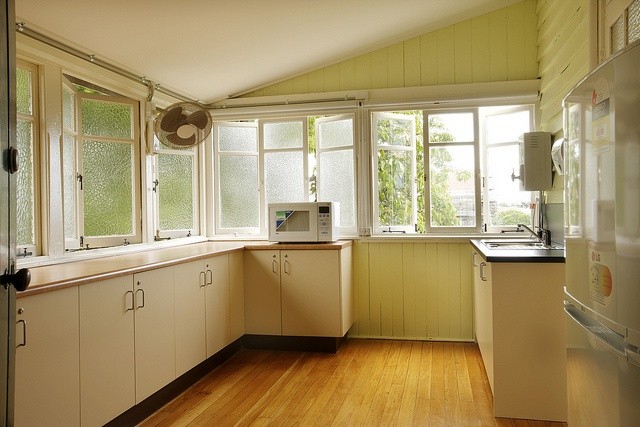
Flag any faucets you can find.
[516,222,553,246]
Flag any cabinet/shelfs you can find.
[471,250,566,422]
[15,285,80,426]
[73,266,177,427]
[174,250,231,392]
[243,243,353,353]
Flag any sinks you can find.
[488,243,547,247]
[485,239,537,245]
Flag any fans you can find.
[154,99,213,148]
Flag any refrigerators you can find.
[560,38,640,427]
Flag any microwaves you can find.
[267,202,340,244]
